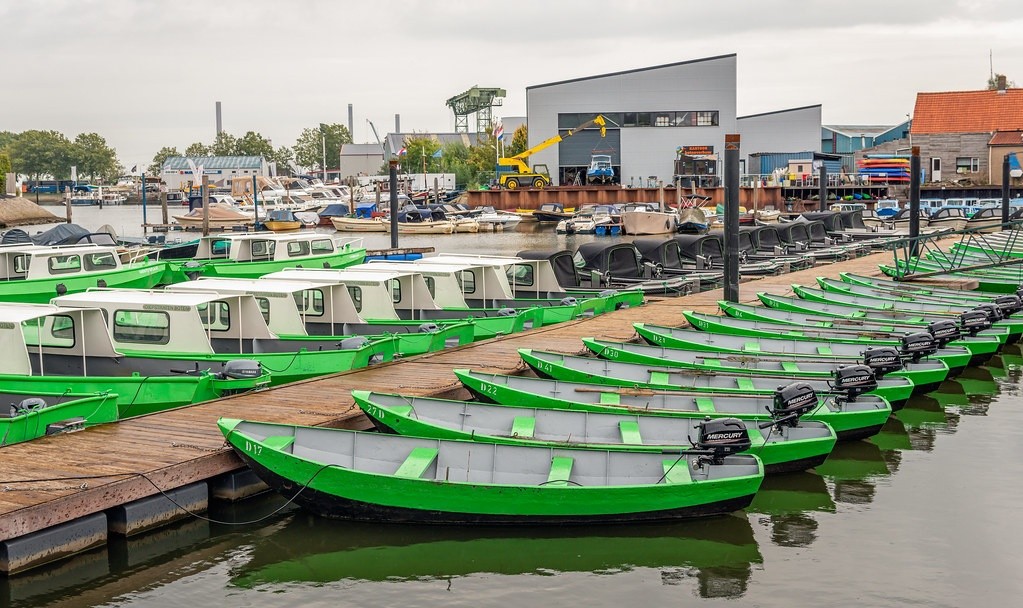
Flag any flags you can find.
[396,147,407,156]
[164,165,171,170]
[71,166,77,181]
[433,148,442,158]
[131,165,137,173]
[492,117,504,140]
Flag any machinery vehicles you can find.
[494,114,608,188]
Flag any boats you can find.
[0,387,122,449]
[347,383,837,477]
[447,361,896,441]
[0,176,1023,414]
[217,413,770,530]
[231,507,766,603]
